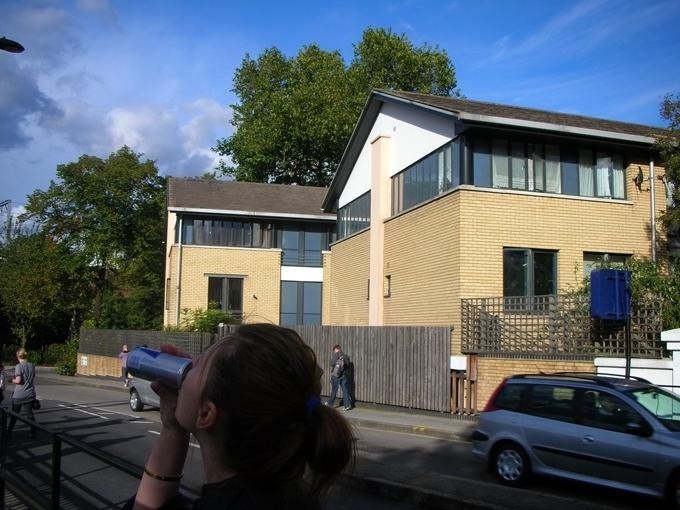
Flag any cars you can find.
[128,376,161,411]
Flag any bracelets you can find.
[143,462,184,481]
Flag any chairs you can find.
[578,391,599,427]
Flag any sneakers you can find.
[124,379,129,388]
[343,407,351,411]
[325,402,329,405]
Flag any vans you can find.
[472,371,680,510]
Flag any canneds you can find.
[125,346,192,391]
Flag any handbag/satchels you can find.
[33,399,40,410]
[331,359,344,378]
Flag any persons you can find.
[7,347,36,443]
[119,344,130,388]
[0,361,6,406]
[119,323,354,510]
[324,344,351,411]
